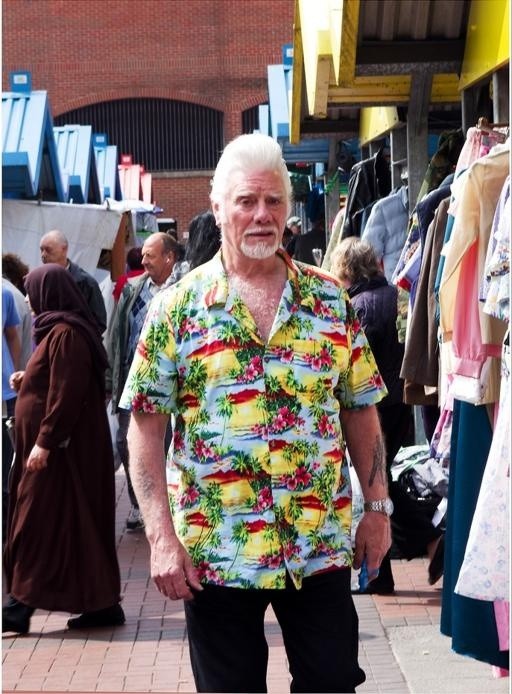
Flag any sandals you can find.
[428,534,445,585]
[351,575,394,595]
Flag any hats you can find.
[287,216,301,227]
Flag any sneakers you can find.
[68,606,125,628]
[2,607,31,633]
[126,509,144,531]
